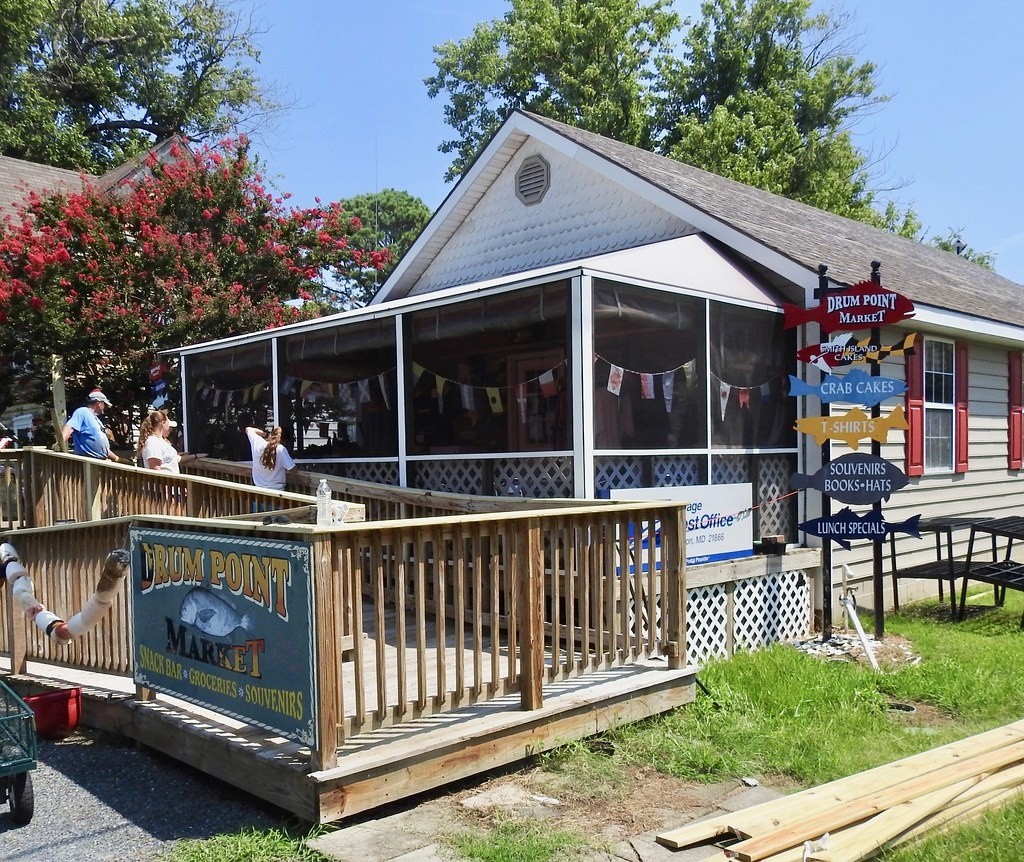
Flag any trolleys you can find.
[0,680,38,819]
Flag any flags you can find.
[413,363,557,424]
[194,381,273,408]
[606,359,699,413]
[279,374,389,410]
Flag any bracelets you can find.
[194,452,197,460]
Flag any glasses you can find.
[263,515,291,525]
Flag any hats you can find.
[88,391,114,406]
[161,414,177,427]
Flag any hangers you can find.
[548,349,632,402]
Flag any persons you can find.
[245,424,298,512]
[138,411,209,503]
[53,391,120,462]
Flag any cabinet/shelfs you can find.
[889,515,1024,630]
